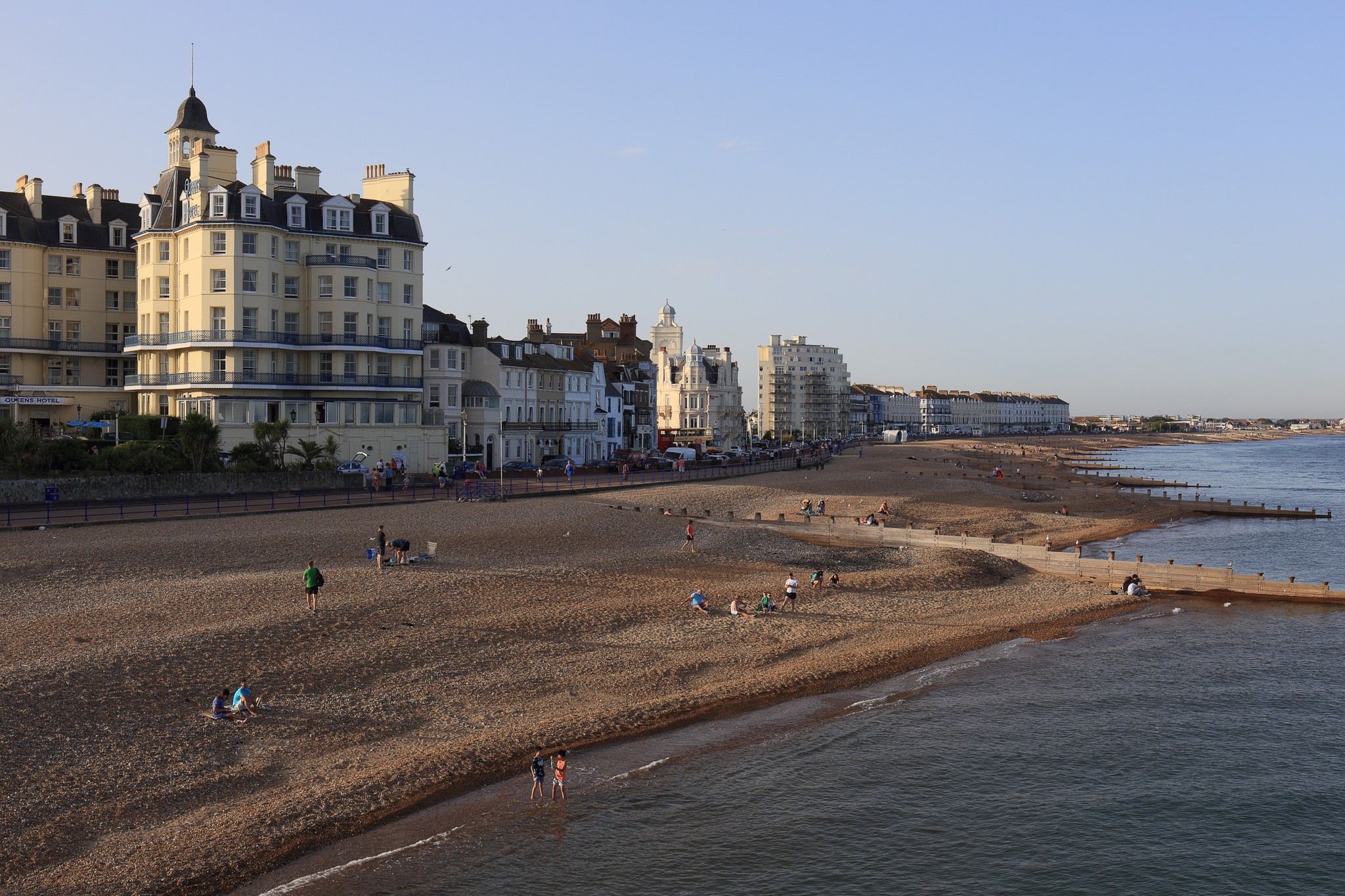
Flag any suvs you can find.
[540,454,572,466]
[101,431,146,441]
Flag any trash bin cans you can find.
[44,485,59,500]
[453,465,460,479]
[434,461,455,478]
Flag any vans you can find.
[662,448,697,467]
[607,450,650,472]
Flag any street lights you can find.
[748,417,754,457]
[460,408,468,481]
[76,404,82,433]
[527,417,532,463]
[779,418,783,450]
[601,418,605,460]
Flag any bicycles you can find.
[536,472,543,481]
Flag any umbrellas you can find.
[65,419,114,438]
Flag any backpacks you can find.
[756,604,762,611]
[315,568,324,587]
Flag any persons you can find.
[614,461,642,478]
[1213,431,1274,437]
[474,460,486,479]
[708,442,711,446]
[536,467,543,478]
[438,461,448,490]
[664,508,840,617]
[92,447,99,456]
[564,461,574,484]
[770,450,780,464]
[531,747,568,798]
[1122,574,1151,596]
[672,457,685,476]
[365,458,410,491]
[302,561,320,614]
[800,499,899,526]
[1147,433,1156,437]
[740,455,746,469]
[910,436,1112,476]
[1055,506,1070,516]
[721,457,728,473]
[793,434,884,471]
[375,525,410,569]
[211,677,260,719]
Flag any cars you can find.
[716,454,731,464]
[218,451,237,469]
[743,417,1121,457]
[334,452,371,475]
[494,461,539,479]
[647,457,670,470]
[1198,425,1302,433]
[539,458,578,476]
[705,456,725,468]
[43,434,88,440]
[451,461,481,477]
[723,451,744,464]
[730,445,744,453]
[581,459,614,474]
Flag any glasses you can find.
[241,683,248,685]
[768,595,772,596]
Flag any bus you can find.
[657,426,724,456]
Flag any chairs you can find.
[417,541,438,565]
[366,549,383,568]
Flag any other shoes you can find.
[813,585,816,588]
[440,487,444,490]
[566,481,572,484]
[674,474,685,476]
[751,614,757,617]
[306,606,312,610]
[313,610,316,613]
[706,611,709,615]
[709,609,716,612]
[262,706,271,711]
[395,564,401,566]
[818,586,821,588]
[761,614,765,616]
[894,512,899,515]
[402,563,407,566]
[481,477,487,479]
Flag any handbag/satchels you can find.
[437,474,439,478]
[367,548,377,560]
[375,475,382,480]
